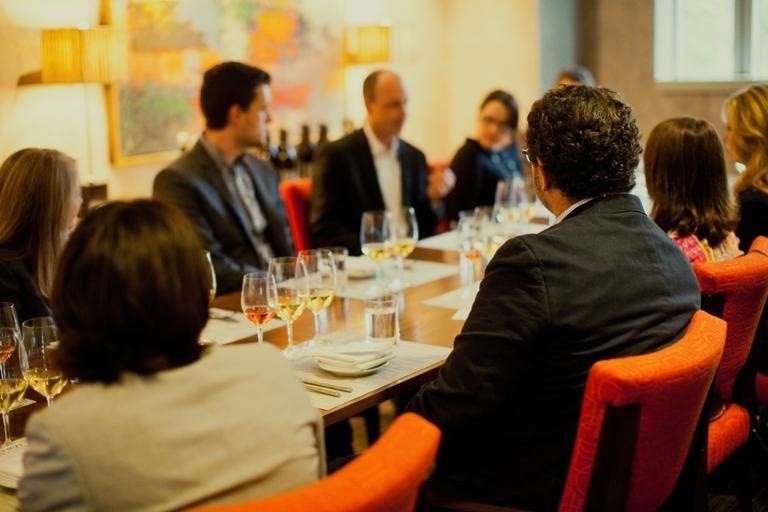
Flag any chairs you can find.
[279,177,313,253]
[429,162,452,234]
[693,235,768,473]
[755,372,768,405]
[176,412,443,512]
[559,310,727,512]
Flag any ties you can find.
[230,167,275,261]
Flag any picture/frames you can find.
[99,0,346,167]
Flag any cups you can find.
[365,297,400,350]
[331,248,348,336]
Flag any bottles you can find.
[315,124,331,157]
[296,124,314,178]
[276,129,294,183]
[259,129,274,166]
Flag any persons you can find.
[308,69,456,250]
[0,149,84,325]
[555,64,595,87]
[437,90,525,227]
[394,84,708,511]
[723,84,767,252]
[644,117,745,261]
[154,61,299,294]
[15,196,327,512]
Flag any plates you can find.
[316,349,391,377]
[319,255,382,282]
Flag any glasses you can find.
[522,147,531,164]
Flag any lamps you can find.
[16,26,117,88]
[341,25,392,67]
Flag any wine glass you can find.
[0,303,22,382]
[204,250,217,310]
[512,175,540,235]
[294,249,337,349]
[384,209,417,314]
[452,211,489,304]
[0,329,29,450]
[241,273,278,345]
[496,181,524,246]
[359,212,398,311]
[266,257,310,359]
[18,316,72,409]
